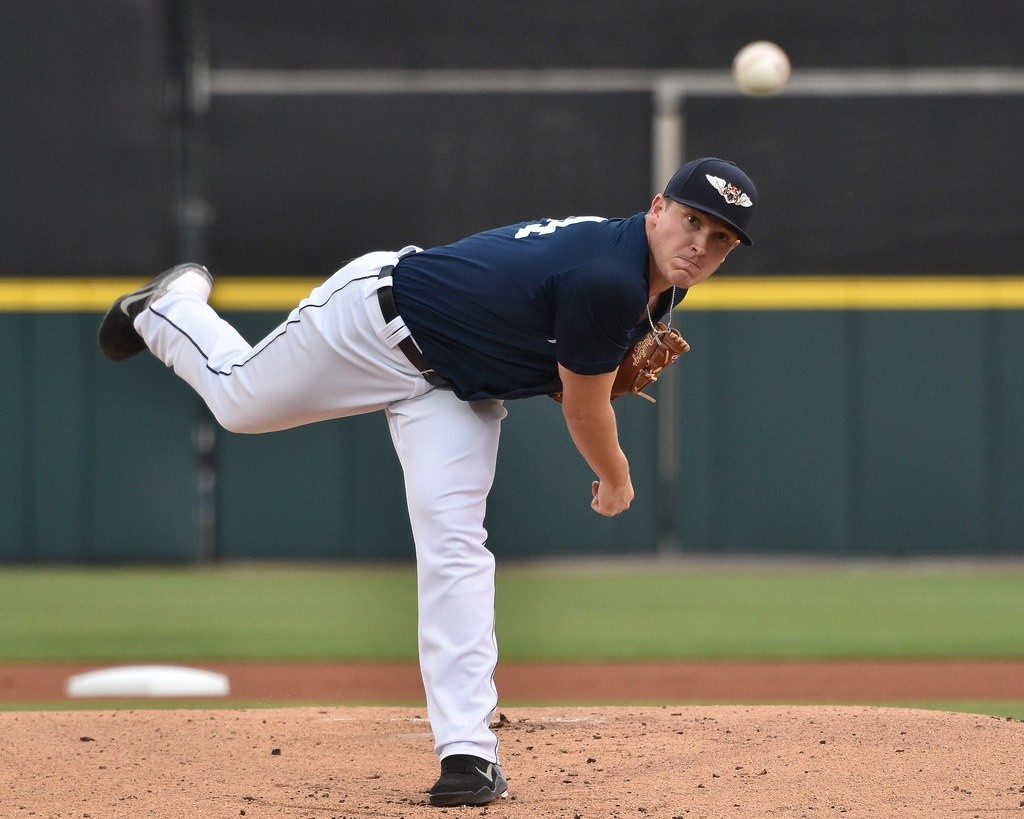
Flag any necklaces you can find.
[646,286,676,335]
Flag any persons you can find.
[97,158,757,806]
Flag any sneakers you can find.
[430,754,509,806]
[98,263,215,361]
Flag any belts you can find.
[377,264,450,389]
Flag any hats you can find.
[663,157,758,247]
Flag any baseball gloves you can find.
[548,320,690,406]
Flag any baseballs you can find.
[730,42,788,99]
